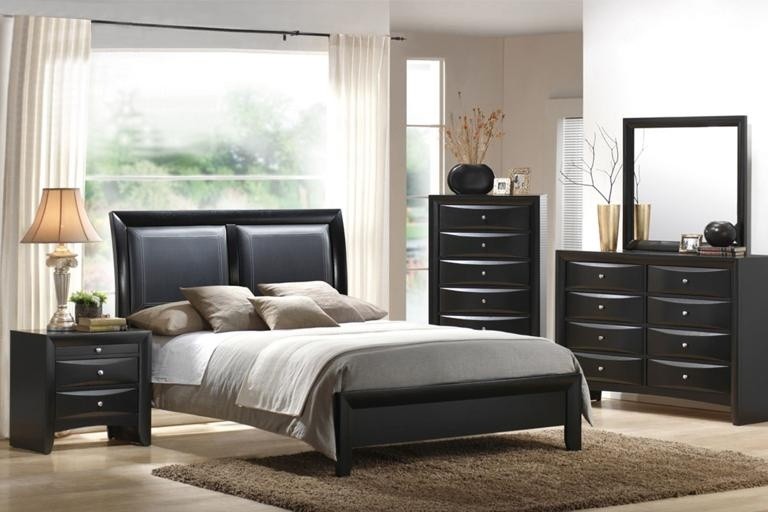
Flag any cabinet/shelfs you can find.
[554,250,768,426]
[429,194,540,337]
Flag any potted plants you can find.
[68,290,107,323]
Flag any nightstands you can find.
[10,329,151,455]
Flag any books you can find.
[699,245,746,256]
[76,316,128,331]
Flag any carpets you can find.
[151,427,768,512]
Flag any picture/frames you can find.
[679,234,703,255]
[492,178,510,196]
[506,168,530,196]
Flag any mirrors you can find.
[623,115,748,253]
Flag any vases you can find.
[597,204,619,252]
[704,221,736,248]
[447,164,495,196]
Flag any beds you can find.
[109,210,594,477]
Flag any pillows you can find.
[126,280,389,335]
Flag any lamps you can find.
[20,188,103,332]
